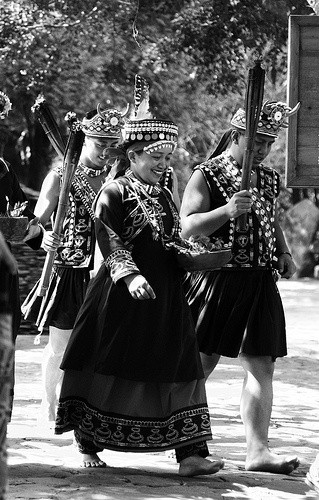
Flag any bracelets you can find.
[276,251,292,257]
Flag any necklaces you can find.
[125,167,181,250]
[227,151,256,175]
[78,161,108,178]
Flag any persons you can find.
[178,100,302,474]
[0,91,45,499]
[21,103,130,423]
[54,118,225,476]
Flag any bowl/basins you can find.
[0,217,28,243]
[184,249,231,271]
